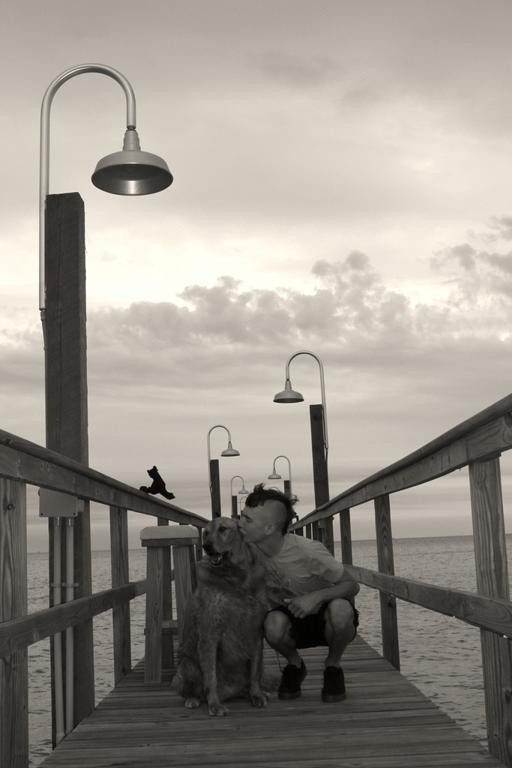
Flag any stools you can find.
[139,525,201,687]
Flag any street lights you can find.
[273,350,333,548]
[207,424,293,518]
[38,62,173,739]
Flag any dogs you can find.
[169,516,278,717]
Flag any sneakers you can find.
[278,658,307,700]
[321,666,345,703]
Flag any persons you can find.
[237,483,360,705]
